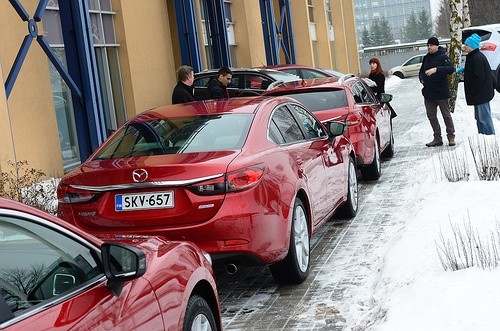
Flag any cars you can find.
[56,95,359,285]
[387,54,429,79]
[0,197,224,331]
[254,65,346,80]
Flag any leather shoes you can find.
[448,138,456,146]
[425,137,443,147]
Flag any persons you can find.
[206,67,232,99]
[172,65,195,104]
[464,33,500,135]
[368,58,397,120]
[419,36,456,147]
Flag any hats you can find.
[427,37,439,46]
[464,33,481,49]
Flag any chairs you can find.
[134,135,240,154]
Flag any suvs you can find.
[189,66,304,103]
[460,23,500,93]
[261,73,395,180]
